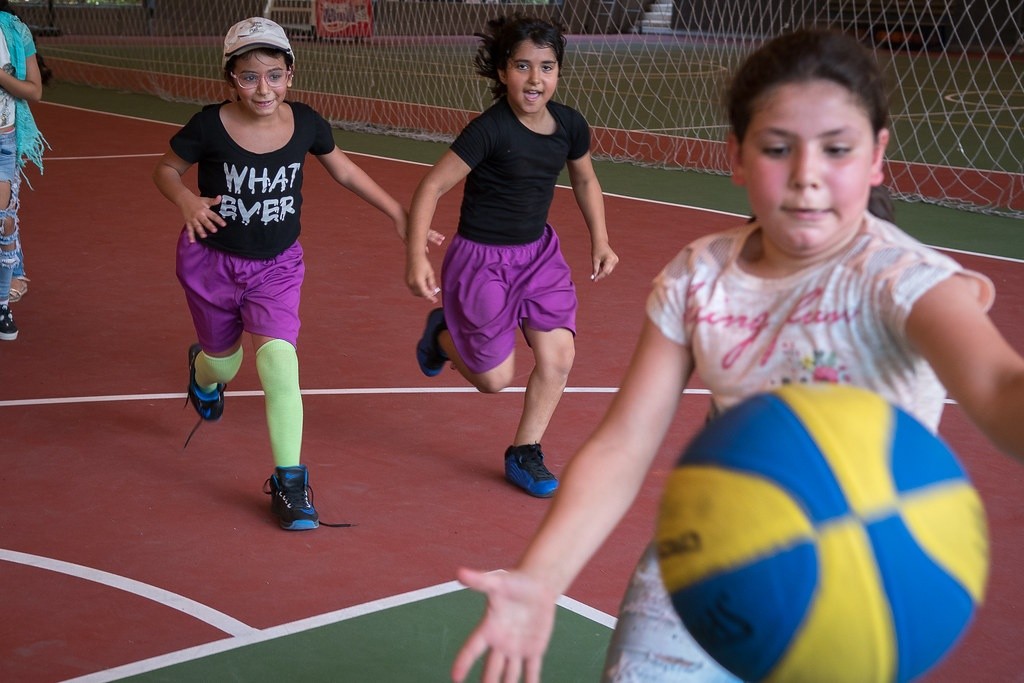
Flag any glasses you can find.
[229,71,293,88]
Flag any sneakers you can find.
[262,465,357,531]
[183,342,229,448]
[417,307,451,377]
[0,303,18,340]
[505,443,558,498]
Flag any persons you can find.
[405,9,618,498]
[0,10,44,343]
[451,28,1024,683]
[154,17,430,532]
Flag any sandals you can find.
[8,276,31,303]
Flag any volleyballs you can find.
[653,384,994,683]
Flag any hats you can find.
[221,18,295,75]
